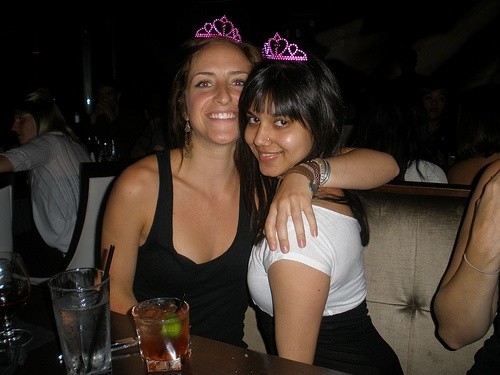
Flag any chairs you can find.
[10,162,127,290]
[0,171,30,275]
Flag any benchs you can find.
[243,181,498,375]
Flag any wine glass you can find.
[0,252,34,352]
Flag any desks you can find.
[110,310,354,375]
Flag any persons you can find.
[238,50,404,375]
[347,79,499,186]
[95,34,399,348]
[1,91,95,285]
[432,159,500,375]
[60,79,176,160]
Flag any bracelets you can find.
[303,159,321,186]
[285,166,319,192]
[315,158,331,187]
[464,253,500,276]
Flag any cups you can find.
[131,297,192,375]
[49,267,113,375]
